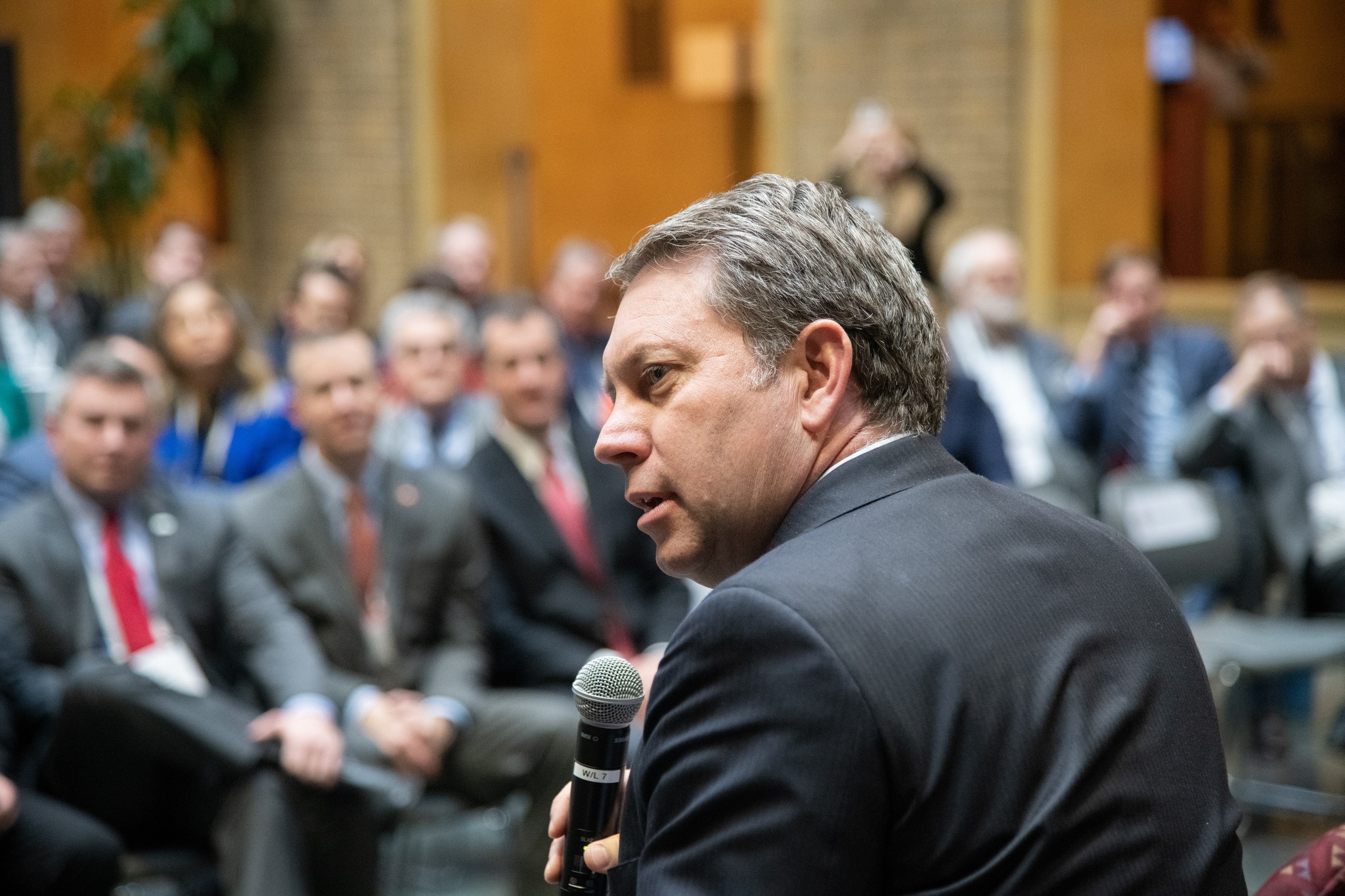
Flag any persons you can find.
[543,166,1252,895]
[829,101,1342,728]
[0,198,687,895]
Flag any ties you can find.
[100,505,157,654]
[536,452,638,661]
[343,481,381,601]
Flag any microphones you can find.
[557,655,646,894]
